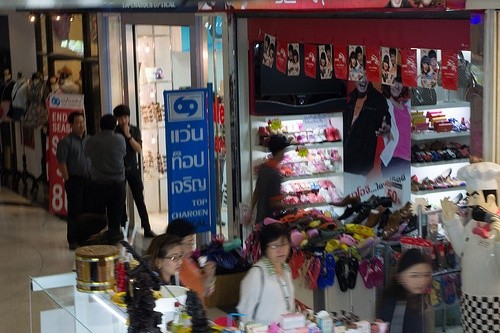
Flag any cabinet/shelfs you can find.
[248,112,344,229]
[131,23,173,179]
[409,100,471,240]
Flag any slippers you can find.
[334,261,348,292]
[288,245,337,290]
[371,257,384,287]
[346,256,359,289]
[359,260,375,288]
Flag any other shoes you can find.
[144,230,157,238]
[410,117,471,192]
[259,126,342,206]
[263,208,381,258]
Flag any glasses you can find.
[160,254,183,261]
[180,241,196,248]
[266,242,291,249]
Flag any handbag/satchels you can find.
[6,103,24,121]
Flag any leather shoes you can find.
[329,194,417,240]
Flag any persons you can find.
[343,46,410,179]
[0,72,87,125]
[376,248,433,333]
[262,35,275,67]
[317,43,333,78]
[235,225,295,325]
[128,218,215,333]
[244,133,292,228]
[287,43,300,75]
[439,161,500,333]
[54,104,158,250]
[420,49,440,87]
